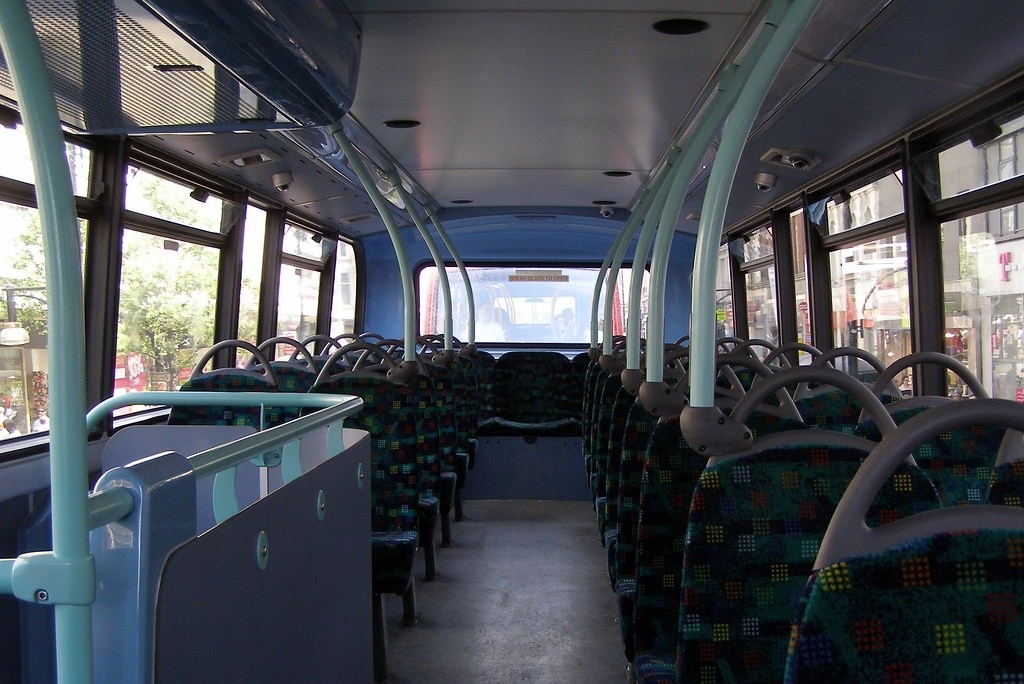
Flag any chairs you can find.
[163,332,1024,684]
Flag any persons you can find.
[897,353,971,402]
[0,398,50,440]
[992,332,1024,358]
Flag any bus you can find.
[420,266,626,345]
